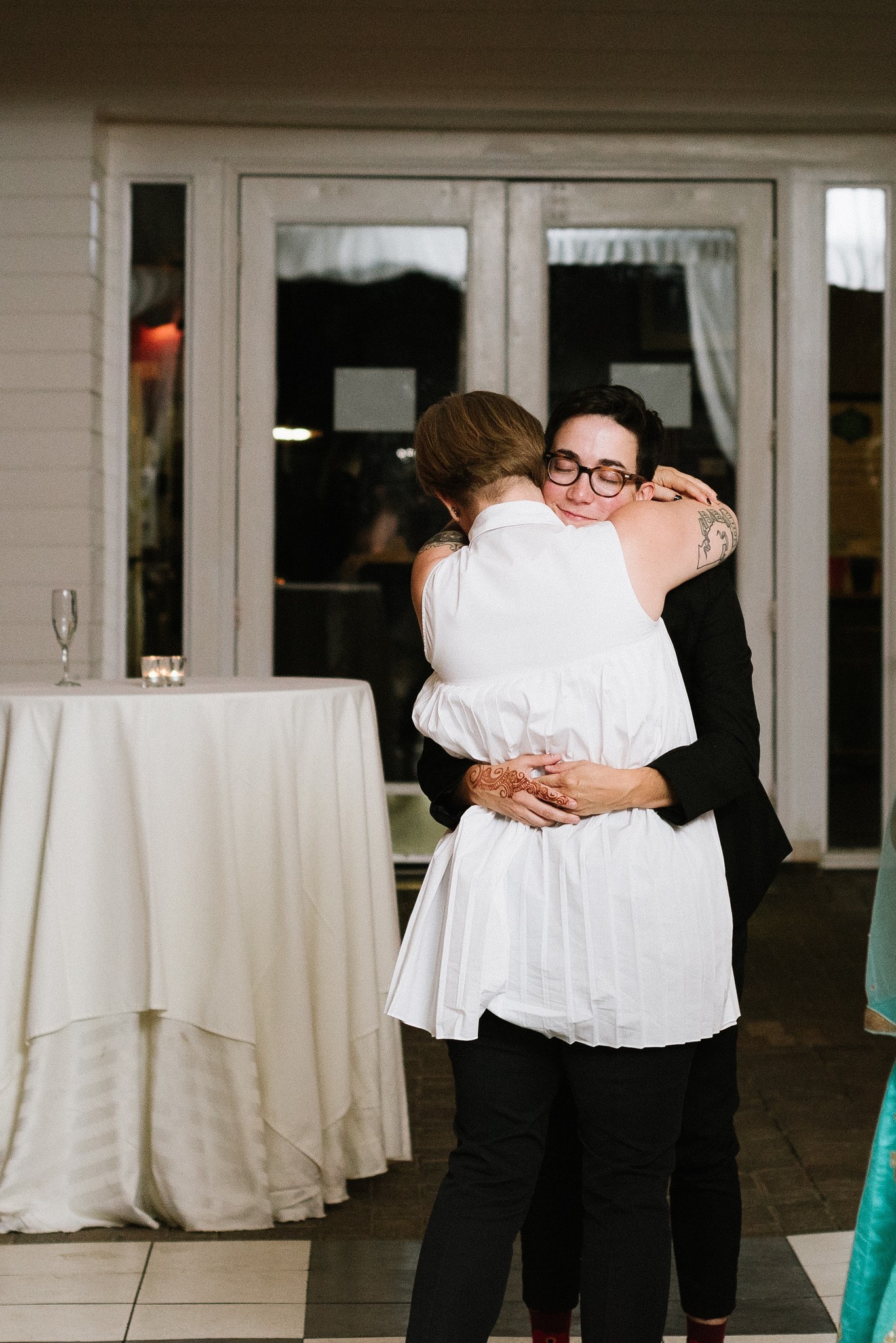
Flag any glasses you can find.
[542,452,647,501]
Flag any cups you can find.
[140,655,186,688]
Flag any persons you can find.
[386,391,738,1343]
[416,383,791,1342]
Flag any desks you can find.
[0,677,412,1234]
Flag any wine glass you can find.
[51,588,81,687]
